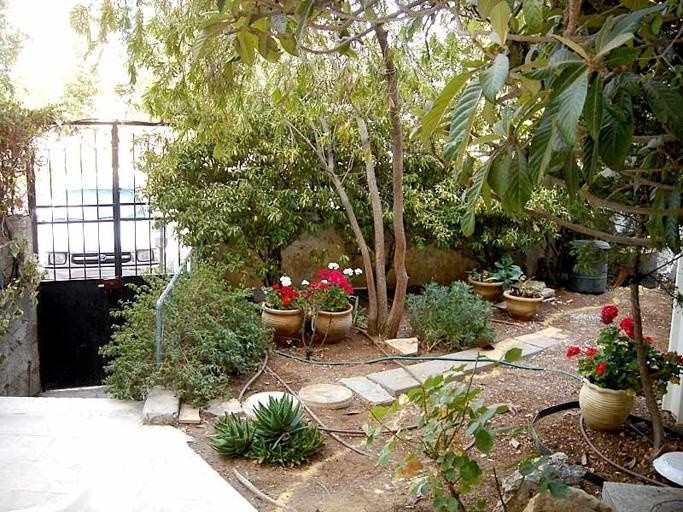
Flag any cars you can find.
[36,187,161,280]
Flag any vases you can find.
[314,302,353,344]
[261,302,303,345]
[578,379,635,430]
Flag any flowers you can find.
[566,305,682,399]
[301,262,362,311]
[265,275,305,311]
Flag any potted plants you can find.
[463,268,505,302]
[502,275,544,320]
